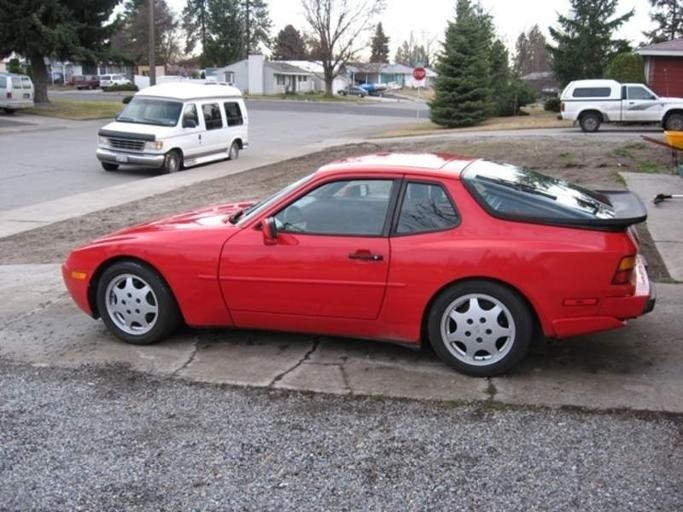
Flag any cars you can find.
[60,149,656,378]
[337,85,368,98]
[65,75,99,90]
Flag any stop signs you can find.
[413,67,426,81]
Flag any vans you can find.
[94,82,249,173]
[99,73,131,89]
[0,72,35,114]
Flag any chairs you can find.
[409,183,430,207]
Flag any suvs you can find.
[558,78,682,132]
